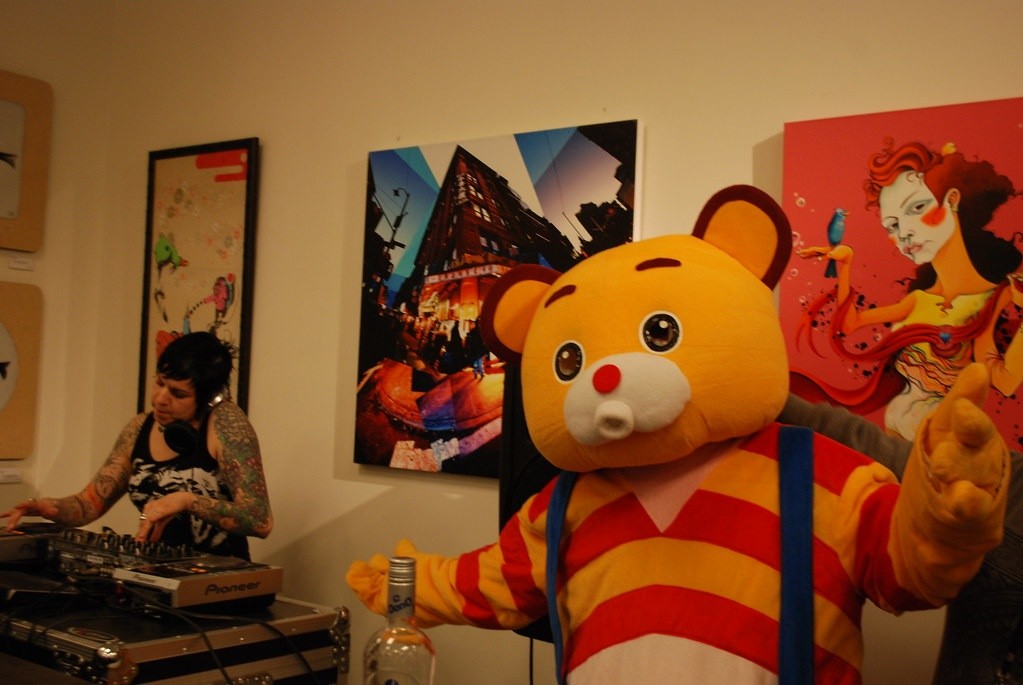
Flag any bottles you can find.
[364,558,436,685]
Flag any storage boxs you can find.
[0,565,351,685]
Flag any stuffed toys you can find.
[344,184,1010,685]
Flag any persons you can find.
[0,331,273,564]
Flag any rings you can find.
[27,498,35,502]
[140,513,146,519]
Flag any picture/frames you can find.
[0,68,55,253]
[0,281,43,460]
[138,137,259,416]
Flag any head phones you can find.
[164,388,230,457]
